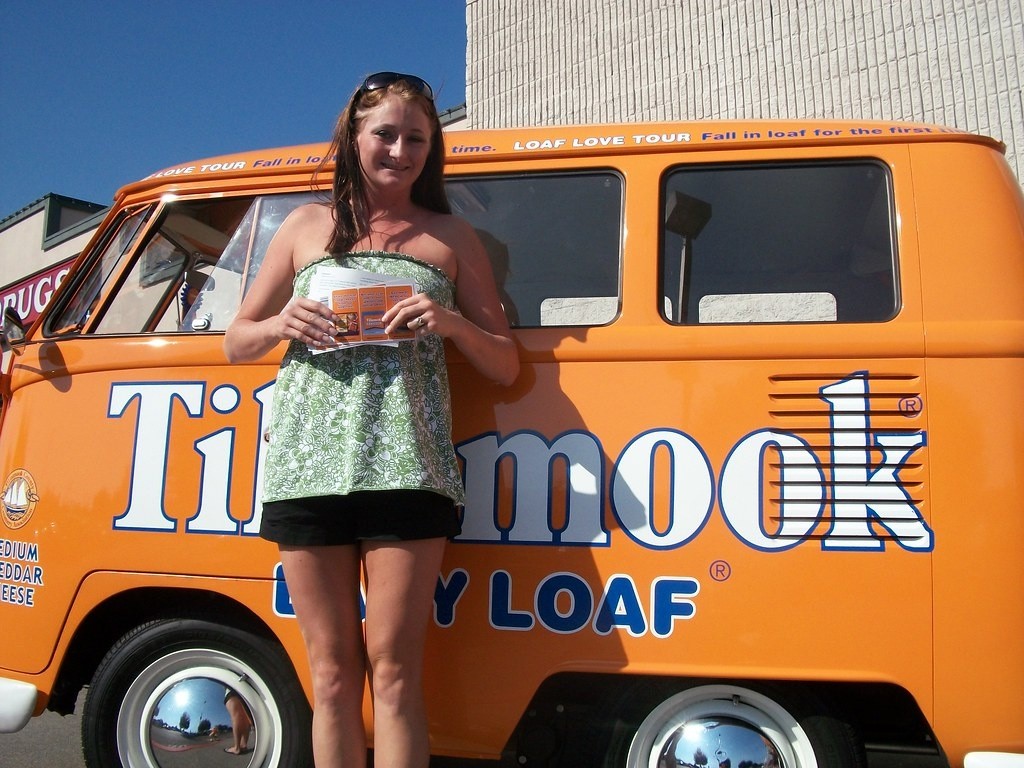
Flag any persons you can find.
[223,72,521,768]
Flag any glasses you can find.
[363,72,434,100]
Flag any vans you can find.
[0,115,1023,768]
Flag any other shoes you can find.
[223,748,233,754]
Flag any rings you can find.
[416,316,424,327]
